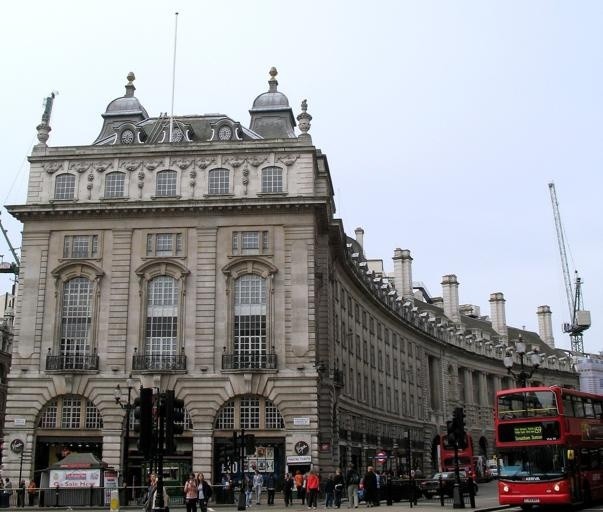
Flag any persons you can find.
[145,471,197,512]
[16,479,24,507]
[0,478,3,507]
[220,465,418,508]
[3,477,12,507]
[27,479,36,506]
[195,472,212,512]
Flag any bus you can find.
[439,432,475,483]
[493,385,602,507]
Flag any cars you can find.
[473,454,501,482]
[420,471,480,499]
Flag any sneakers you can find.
[245,503,375,510]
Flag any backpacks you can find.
[349,472,359,485]
[207,483,212,498]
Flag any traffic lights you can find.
[130,386,146,446]
[218,430,254,467]
[170,391,186,449]
[445,405,469,445]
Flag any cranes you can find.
[546,183,594,359]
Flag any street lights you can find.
[501,333,544,419]
[112,373,145,505]
[392,427,414,509]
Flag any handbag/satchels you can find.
[334,483,343,491]
[142,494,149,504]
[143,497,153,512]
